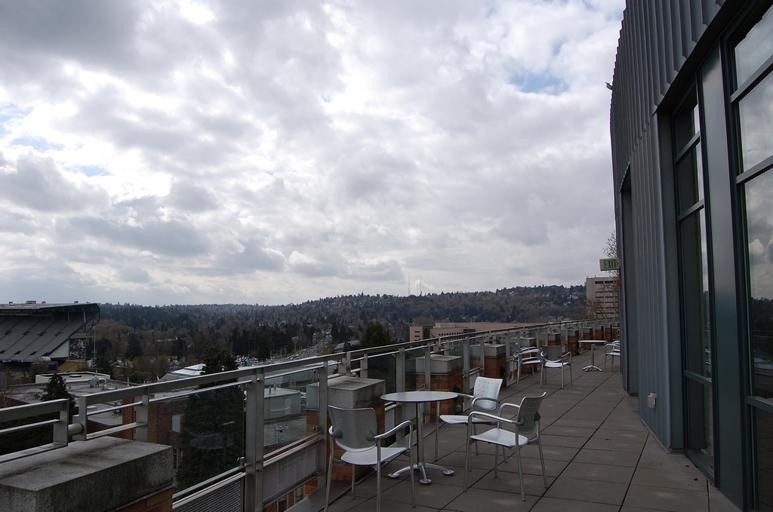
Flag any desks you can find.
[379,388,458,486]
[579,339,607,373]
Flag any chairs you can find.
[438,374,507,478]
[512,341,541,385]
[323,403,415,512]
[538,344,574,390]
[604,336,620,369]
[463,391,549,502]
